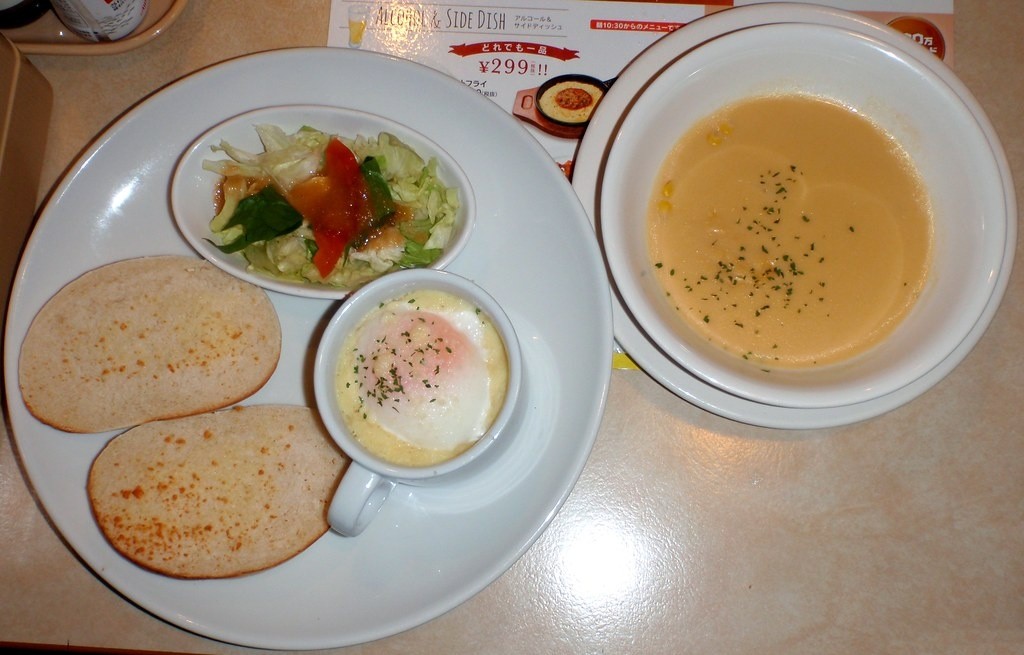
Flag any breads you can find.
[18,254,283,434]
[86,404,352,579]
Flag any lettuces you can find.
[200,122,461,289]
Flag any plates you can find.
[0,0,186,54]
[570,5,1018,430]
[3,47,615,651]
[170,104,476,301]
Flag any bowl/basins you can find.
[599,23,1008,410]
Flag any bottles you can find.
[51,0,149,41]
[0,0,51,30]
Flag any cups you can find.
[313,267,529,538]
